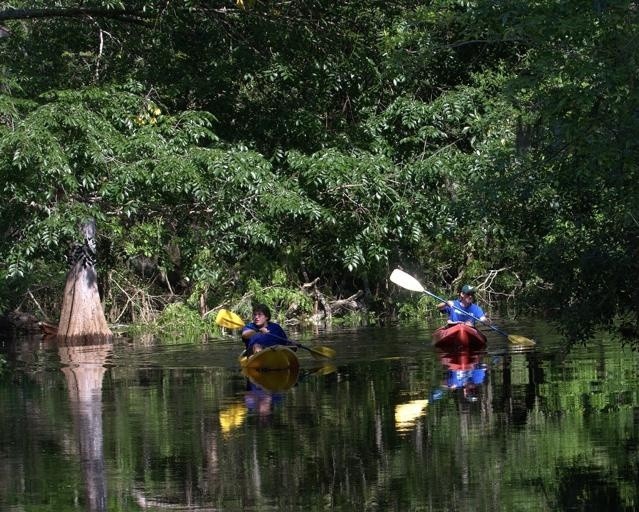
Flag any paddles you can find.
[390,269,535,347]
[215,310,337,362]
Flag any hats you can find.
[462,284,476,295]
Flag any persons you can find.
[440,363,486,403]
[242,305,287,358]
[244,383,287,417]
[438,284,487,328]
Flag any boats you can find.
[238,346,302,370]
[243,367,302,394]
[439,348,484,374]
[430,321,491,350]
